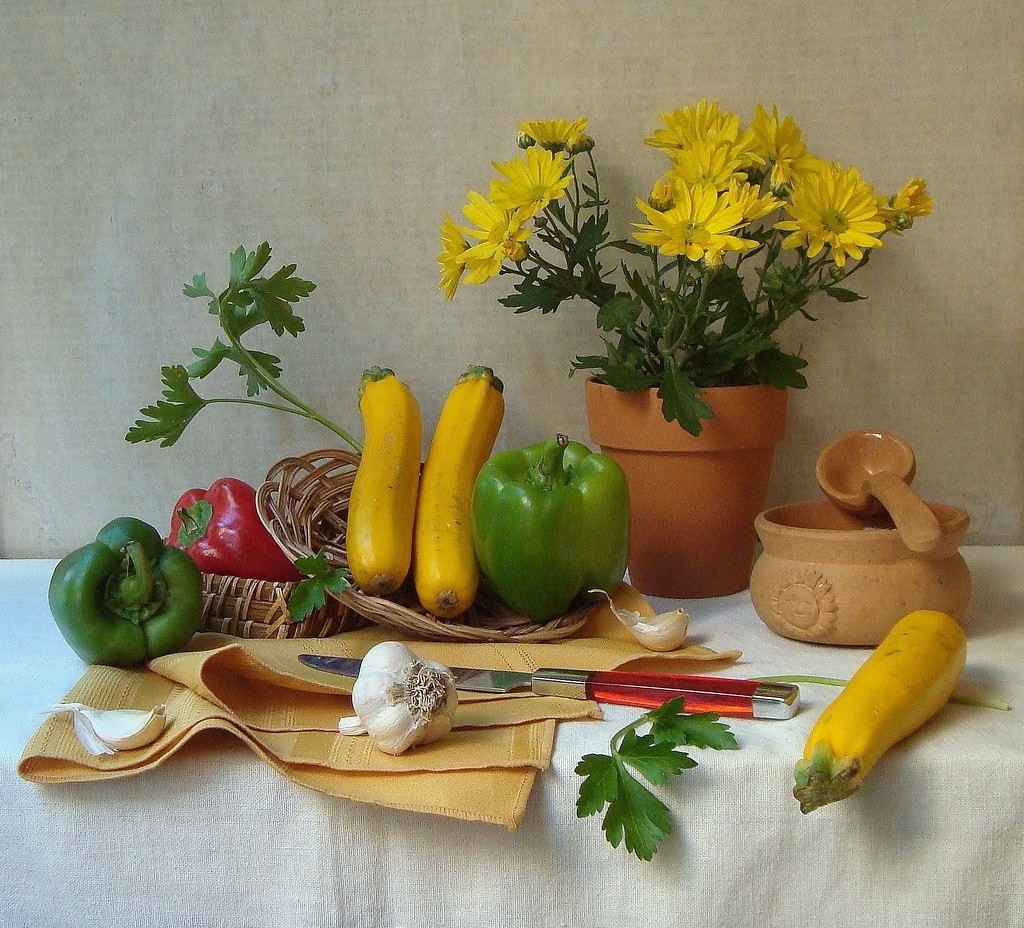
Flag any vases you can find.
[582,376,790,603]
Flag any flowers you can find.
[432,82,932,441]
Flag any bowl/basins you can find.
[750,500,971,646]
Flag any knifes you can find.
[299,653,802,721]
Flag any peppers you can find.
[43,515,204,670]
[167,479,304,584]
[471,426,638,625]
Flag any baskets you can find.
[195,570,376,640]
[253,447,591,644]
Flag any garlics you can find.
[337,642,456,756]
[586,582,689,649]
[37,702,166,756]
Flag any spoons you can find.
[816,431,947,552]
